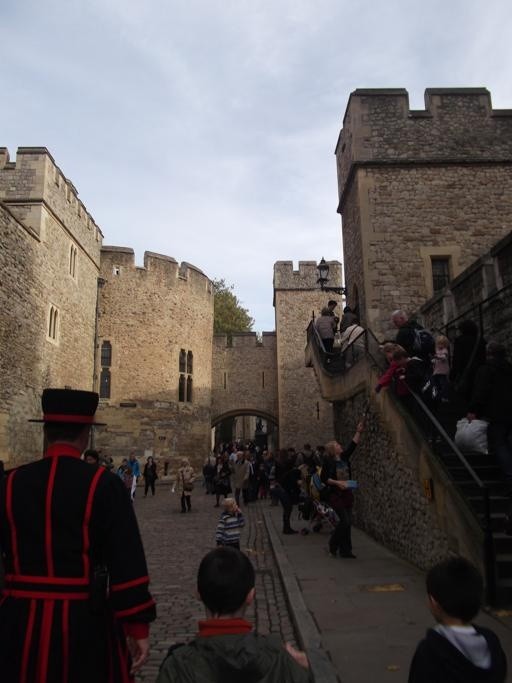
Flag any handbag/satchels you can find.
[267,481,287,501]
[341,339,352,362]
[454,418,489,456]
[182,482,195,491]
[333,338,341,347]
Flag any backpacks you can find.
[413,329,435,357]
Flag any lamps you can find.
[315,257,347,296]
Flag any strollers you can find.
[302,484,341,536]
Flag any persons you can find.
[156,544,315,682]
[374,308,493,438]
[1,387,157,681]
[95,447,196,513]
[315,299,365,370]
[408,556,508,683]
[201,421,364,556]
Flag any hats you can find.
[27,388,108,425]
